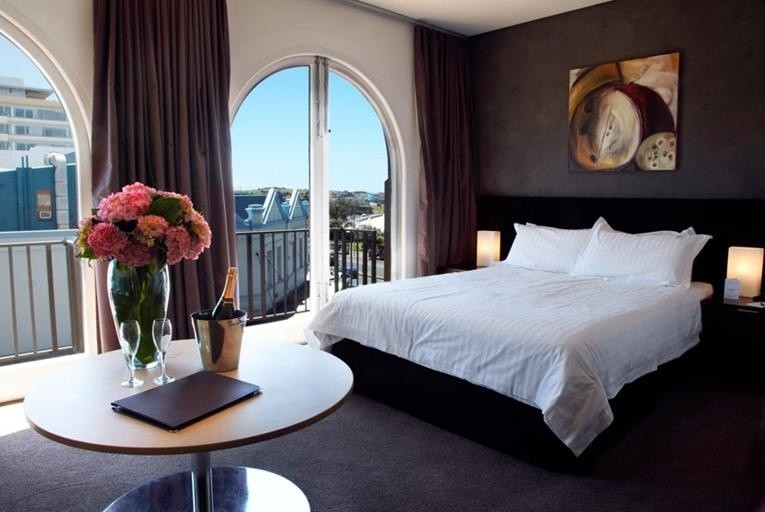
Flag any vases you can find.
[106,258,171,369]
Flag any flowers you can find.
[74,182,213,358]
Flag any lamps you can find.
[476,230,501,267]
[726,247,764,298]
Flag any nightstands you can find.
[700,293,765,386]
[439,260,476,271]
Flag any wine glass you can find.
[120,320,146,388]
[152,321,176,383]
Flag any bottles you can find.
[214,266,237,322]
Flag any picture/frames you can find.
[566,50,680,173]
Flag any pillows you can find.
[502,216,713,288]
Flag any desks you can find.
[23,338,356,512]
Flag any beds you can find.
[306,195,765,476]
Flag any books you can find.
[110,368,264,434]
